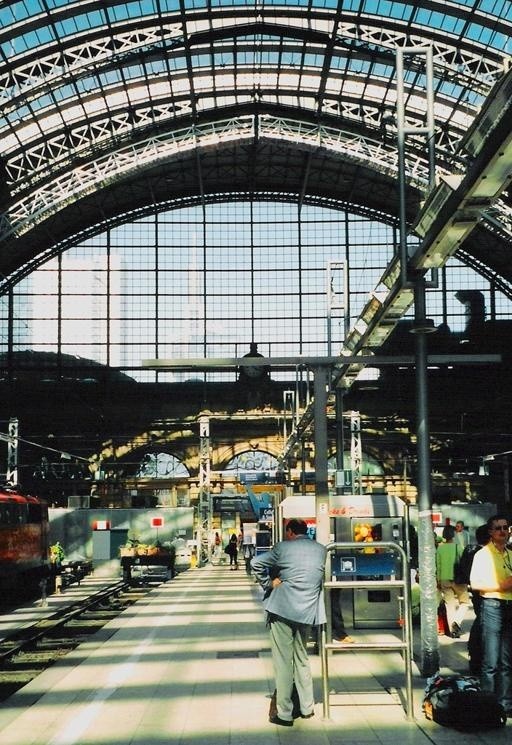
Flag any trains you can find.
[0,489,52,585]
[275,494,418,543]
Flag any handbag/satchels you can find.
[460,545,482,584]
[269,682,298,725]
[422,675,507,729]
[454,563,468,585]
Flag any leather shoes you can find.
[272,716,293,726]
[301,711,314,718]
[451,622,464,638]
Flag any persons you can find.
[407,513,512,719]
[250,517,327,726]
[227,533,254,572]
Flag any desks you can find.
[119,551,171,581]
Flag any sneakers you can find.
[332,637,353,644]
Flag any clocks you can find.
[236,342,271,391]
